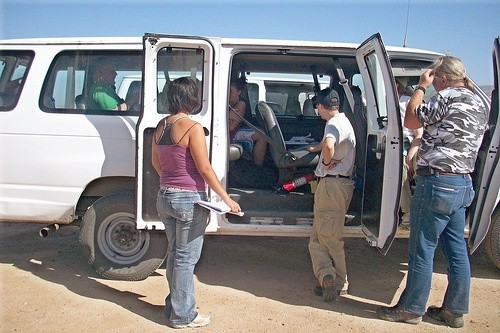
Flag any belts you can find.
[325,173,350,178]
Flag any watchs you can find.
[415,85,426,95]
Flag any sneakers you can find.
[171,313,209,329]
[426,304,465,328]
[376,306,422,324]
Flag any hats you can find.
[312,88,341,105]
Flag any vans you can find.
[0,32,500,282]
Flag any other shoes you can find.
[314,286,347,296]
[400,212,411,230]
[321,275,338,303]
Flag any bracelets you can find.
[321,159,332,166]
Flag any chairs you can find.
[255,102,321,192]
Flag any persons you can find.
[228,77,274,186]
[377,55,488,328]
[395,76,423,234]
[307,87,357,301]
[151,77,242,328]
[85,56,139,112]
